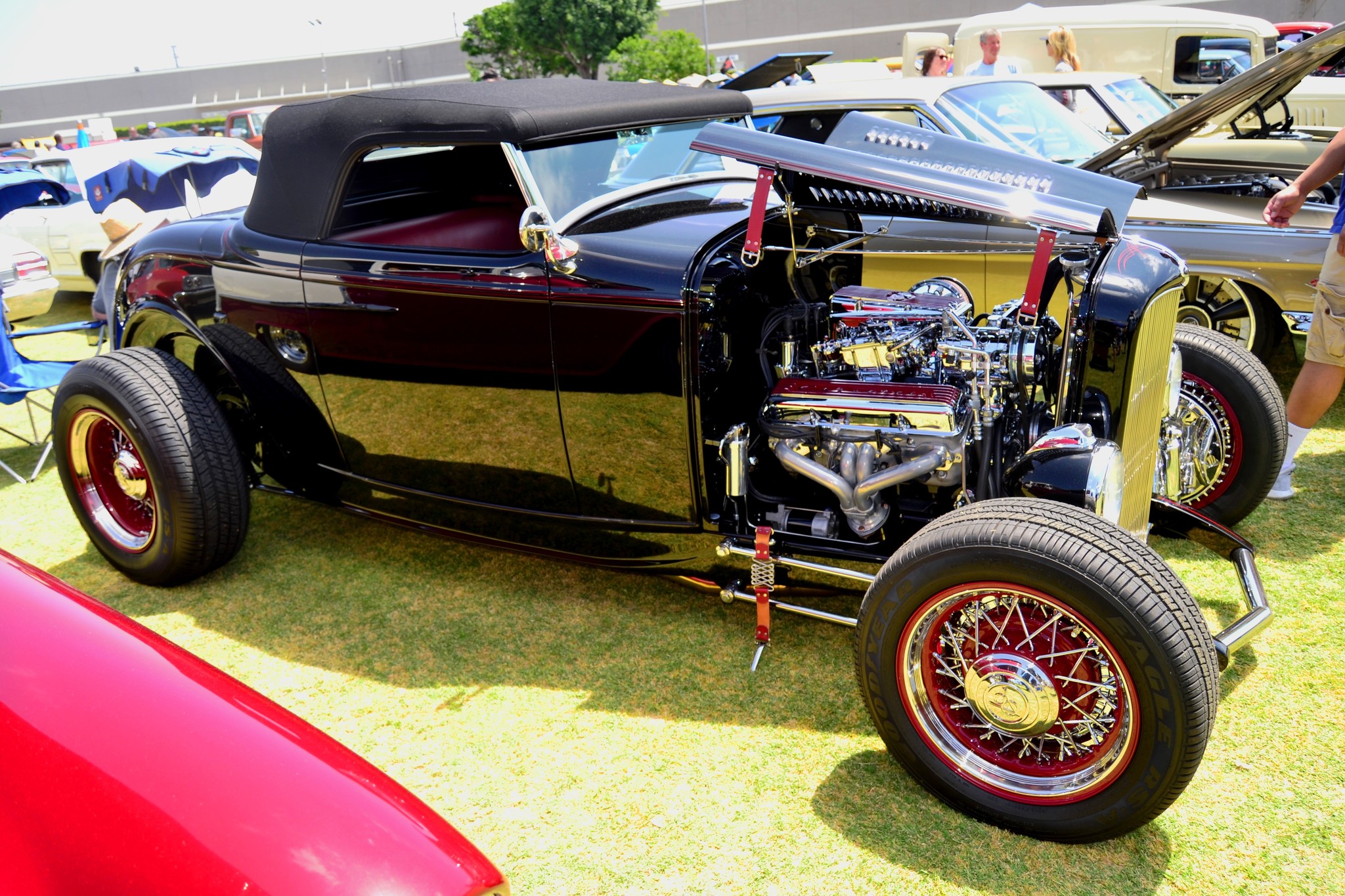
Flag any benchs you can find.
[325,207,521,256]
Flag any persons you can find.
[52,134,72,152]
[33,140,47,157]
[92,198,166,353]
[146,122,168,139]
[127,126,147,140]
[188,123,199,136]
[1039,26,1080,114]
[1263,127,1345,499]
[963,27,1028,75]
[921,45,948,76]
[10,141,22,149]
[202,127,215,137]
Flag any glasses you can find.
[1046,39,1049,46]
[937,54,948,60]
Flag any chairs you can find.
[0,281,106,485]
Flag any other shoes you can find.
[1266,463,1296,500]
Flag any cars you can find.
[1,548,515,896]
[0,2,1345,377]
[50,78,1291,849]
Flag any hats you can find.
[1040,34,1051,44]
[96,198,169,263]
